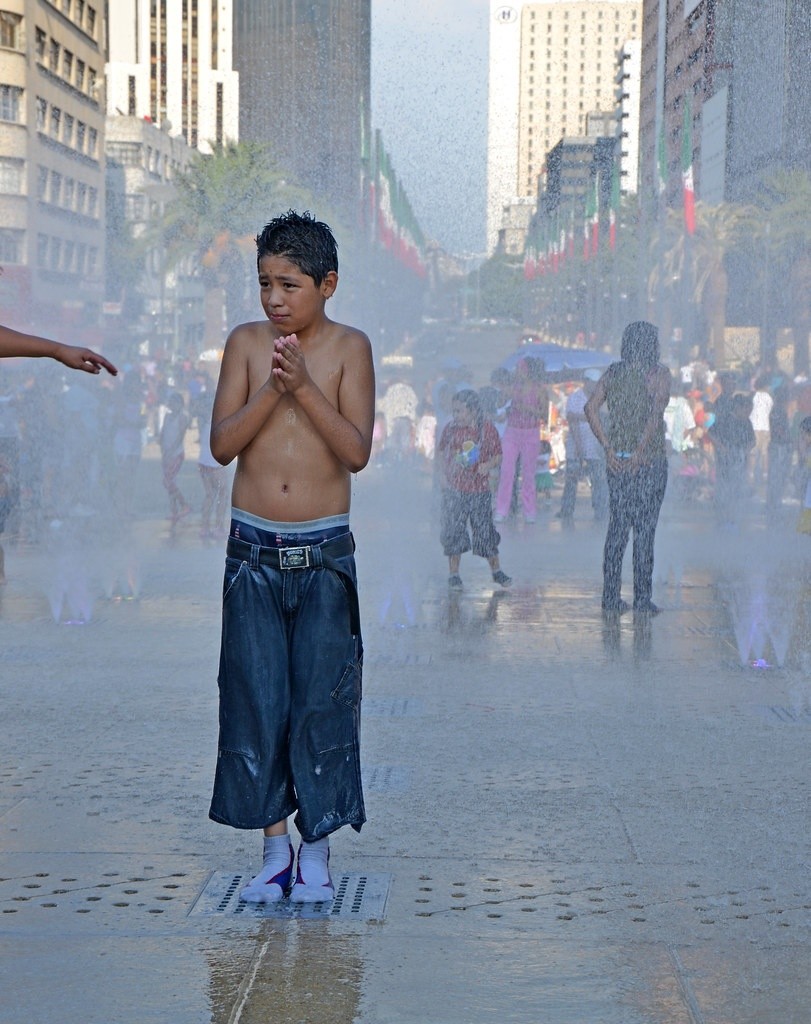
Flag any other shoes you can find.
[448,576,463,590]
[524,517,536,524]
[494,512,506,522]
[634,602,657,613]
[555,510,573,519]
[602,600,627,612]
[493,570,512,587]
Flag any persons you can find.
[208,213,375,903]
[586,321,672,614]
[361,358,811,590]
[0,324,240,538]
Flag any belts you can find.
[226,538,350,570]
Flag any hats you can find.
[584,369,602,381]
